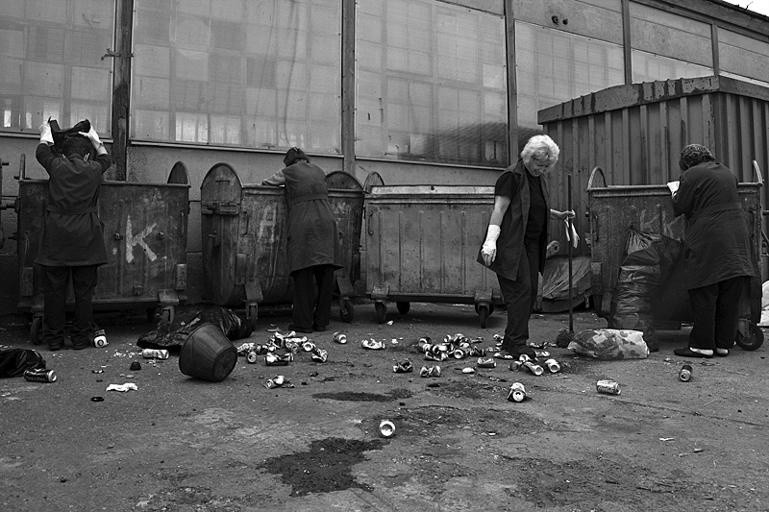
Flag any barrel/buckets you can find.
[179,322,238,379]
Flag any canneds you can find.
[597,380,622,395]
[237,330,328,388]
[359,332,559,403]
[333,332,347,344]
[679,365,693,382]
[24,369,56,381]
[379,420,395,438]
[143,348,169,359]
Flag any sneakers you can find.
[501,339,535,359]
[288,325,326,334]
[48,339,91,350]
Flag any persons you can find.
[666,142,755,361]
[477,132,577,361]
[33,112,112,352]
[261,145,346,334]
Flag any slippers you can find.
[673,345,731,358]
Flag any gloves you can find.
[564,216,581,250]
[78,123,104,152]
[481,225,501,267]
[666,180,680,198]
[38,120,56,147]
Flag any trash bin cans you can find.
[199,161,364,329]
[7,153,191,345]
[361,171,505,328]
[586,160,764,352]
